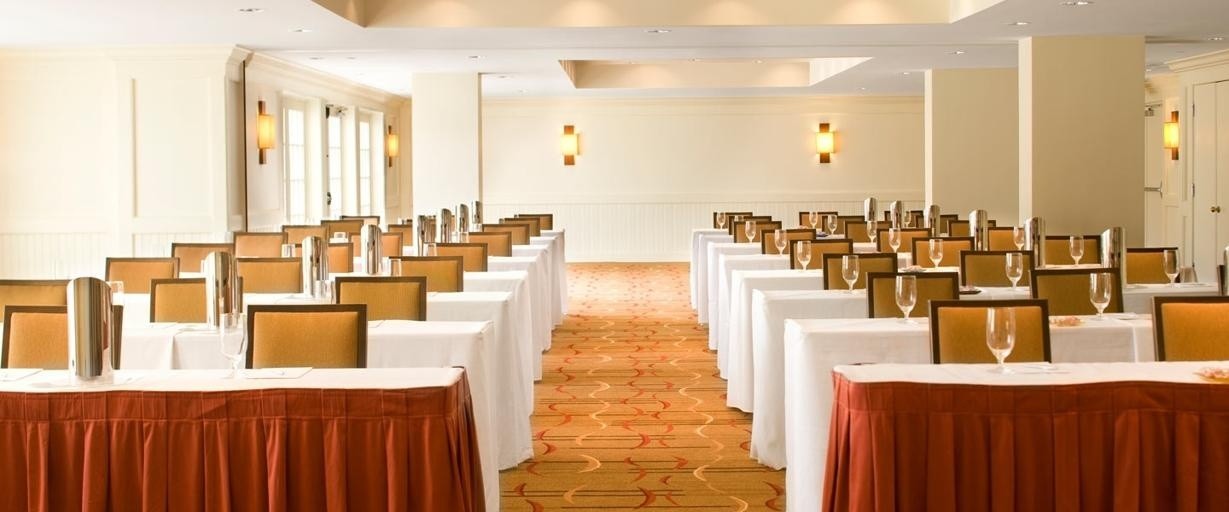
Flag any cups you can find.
[107,280,124,306]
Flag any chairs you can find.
[1,202,570,368]
[690,207,1229,364]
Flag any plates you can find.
[959,289,982,294]
[1049,319,1085,327]
[1192,371,1229,382]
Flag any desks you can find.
[0,367,488,512]
[819,364,1229,512]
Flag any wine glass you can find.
[1006,252,1024,291]
[1161,249,1183,289]
[1069,234,1087,268]
[1087,270,1114,326]
[712,202,945,274]
[1010,225,1028,252]
[216,221,488,375]
[895,274,920,325]
[839,254,861,296]
[984,305,1017,378]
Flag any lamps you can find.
[387,126,400,167]
[257,99,277,166]
[1162,111,1180,162]
[815,123,835,163]
[561,125,579,166]
